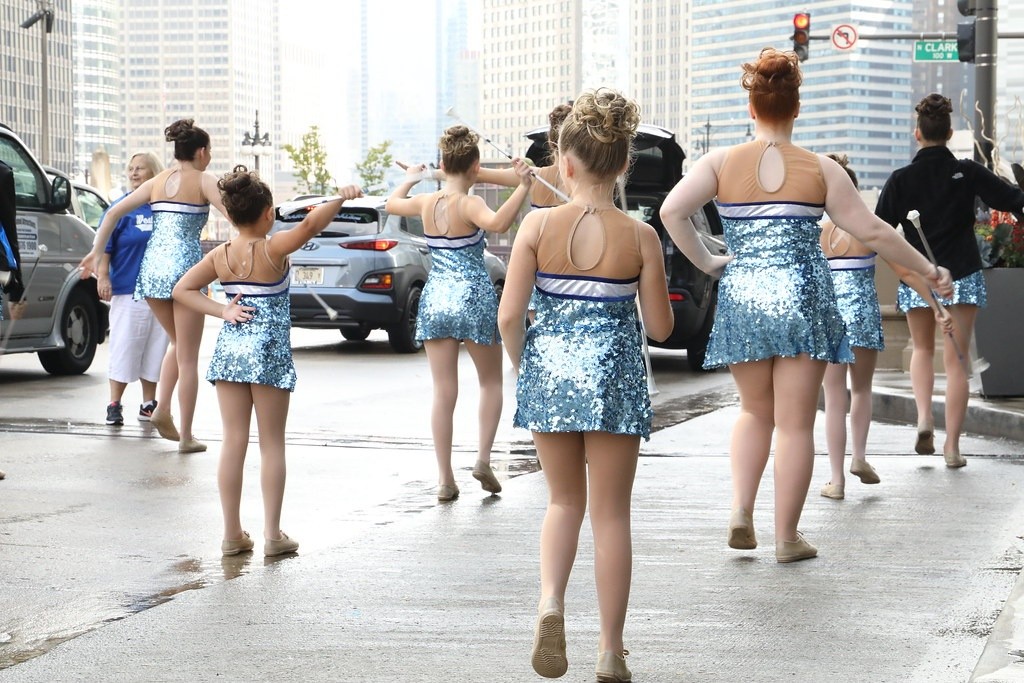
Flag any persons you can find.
[78,118,236,453]
[0,159,27,320]
[171,165,365,557]
[393,104,573,326]
[875,94,1024,468]
[496,87,674,683]
[97,151,169,423]
[818,153,955,499]
[384,126,533,500]
[659,47,954,562]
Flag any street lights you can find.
[20,3,55,164]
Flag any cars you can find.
[13,165,113,232]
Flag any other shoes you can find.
[915,427,935,454]
[265,530,298,556]
[439,481,459,501]
[179,436,206,453]
[821,479,845,499]
[531,607,569,678]
[945,454,967,468]
[851,460,880,484]
[596,650,632,682]
[776,530,817,563]
[151,404,181,440]
[221,528,254,555]
[728,509,756,548]
[473,459,501,492]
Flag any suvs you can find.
[0,121,112,377]
[525,121,726,373]
[264,194,506,353]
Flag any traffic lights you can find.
[793,13,811,61]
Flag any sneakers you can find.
[138,401,173,422]
[106,402,123,425]
